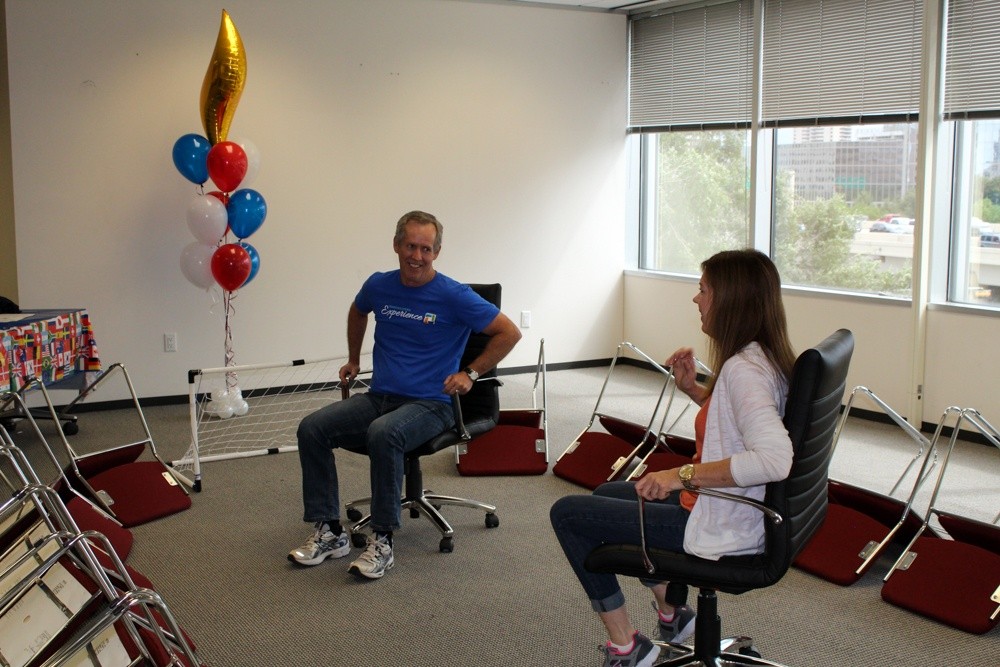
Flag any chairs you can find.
[553,327,1000,667]
[345,283,504,553]
[0,364,196,667]
[454,338,549,475]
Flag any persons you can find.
[464,367,478,382]
[287,210,522,580]
[550,249,796,667]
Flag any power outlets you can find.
[521,311,530,327]
[165,332,176,352]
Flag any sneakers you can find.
[287,521,351,566]
[349,533,395,579]
[597,629,662,667]
[651,600,697,644]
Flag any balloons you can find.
[205,385,249,418]
[172,9,267,293]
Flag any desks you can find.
[0,308,103,436]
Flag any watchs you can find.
[679,464,699,490]
